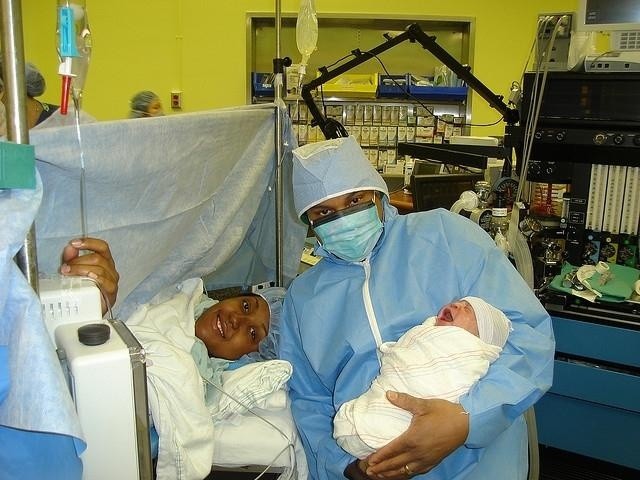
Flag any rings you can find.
[405,464,416,476]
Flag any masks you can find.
[307,191,386,261]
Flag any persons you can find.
[0,52,97,138]
[129,90,162,118]
[277,137,555,480]
[332,295,511,459]
[60,238,286,429]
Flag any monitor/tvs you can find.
[410,173,484,215]
[577,1,640,32]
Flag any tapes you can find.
[595,261,610,274]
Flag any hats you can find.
[291,136,389,225]
[460,296,514,347]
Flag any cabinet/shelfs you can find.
[244,11,476,180]
[530,303,639,471]
